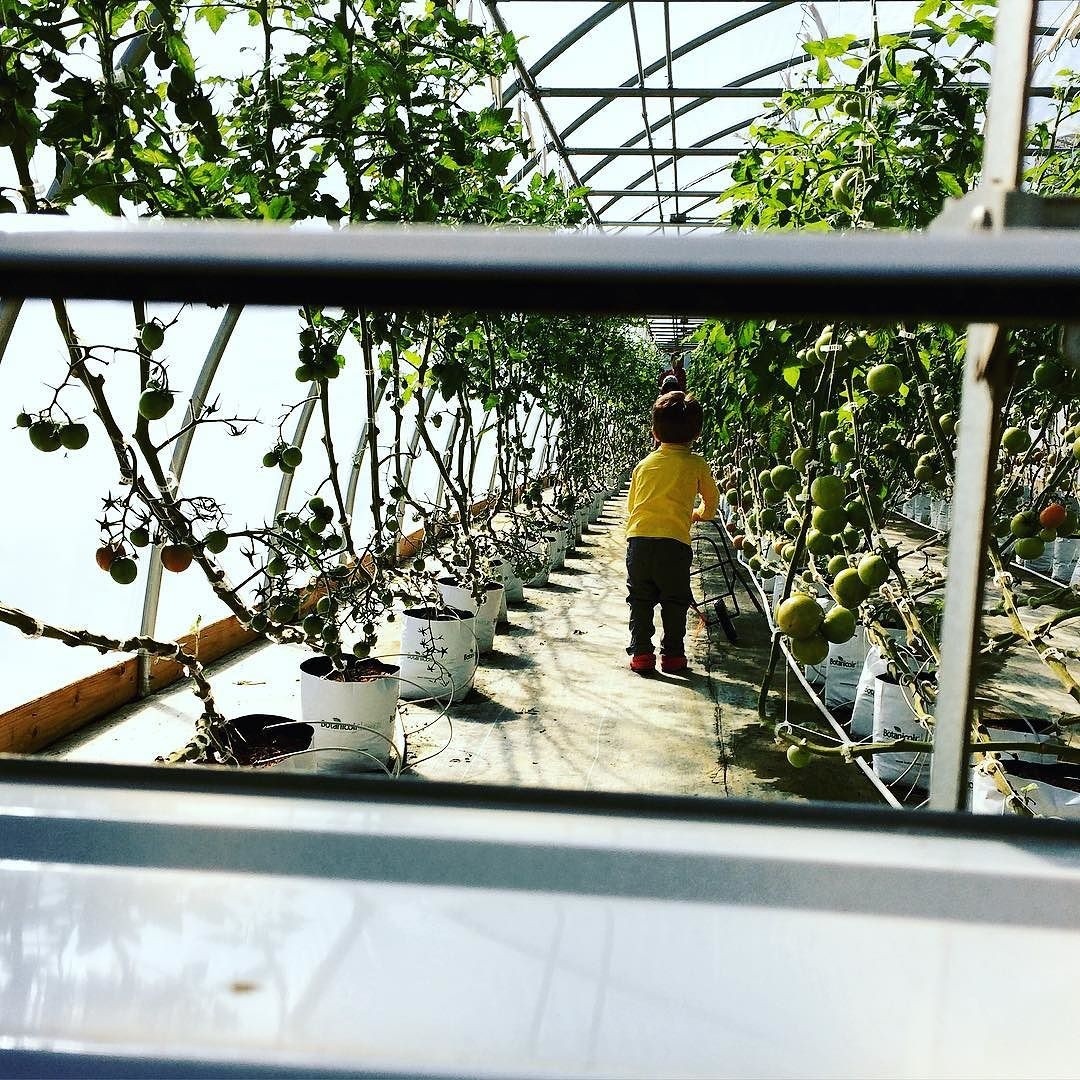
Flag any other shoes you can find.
[630,653,656,672]
[661,654,687,673]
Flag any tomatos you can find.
[0,1,635,665]
[690,95,1080,770]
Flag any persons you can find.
[657,358,685,394]
[624,390,721,673]
[654,375,685,450]
